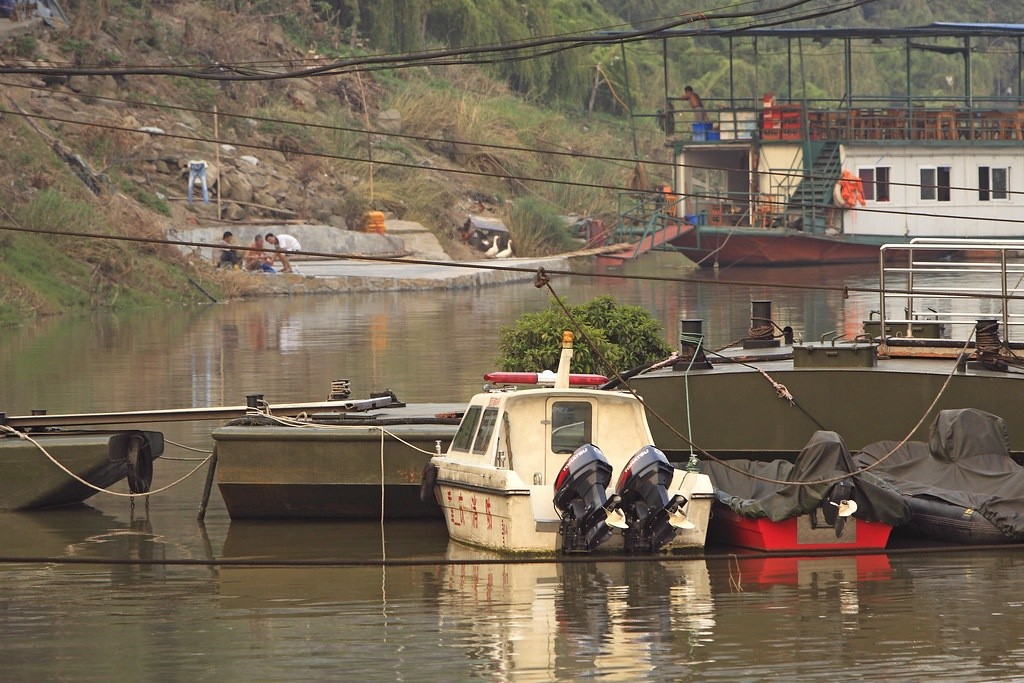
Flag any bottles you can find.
[784,116,798,135]
[711,206,720,222]
[764,128,776,136]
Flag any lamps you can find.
[812,33,821,43]
[872,37,883,44]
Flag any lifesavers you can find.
[832,179,859,210]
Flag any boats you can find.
[594,22,1024,269]
[730,552,894,589]
[0,426,167,515]
[211,238,1023,523]
[672,430,902,551]
[854,407,1024,545]
[428,330,716,555]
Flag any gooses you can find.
[483,235,513,258]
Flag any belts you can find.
[693,105,703,108]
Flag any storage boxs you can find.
[708,203,732,226]
[685,213,705,227]
[762,95,801,139]
[691,121,719,142]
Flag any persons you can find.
[213,231,301,273]
[680,86,708,121]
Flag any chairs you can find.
[810,107,1024,139]
[752,195,777,228]
